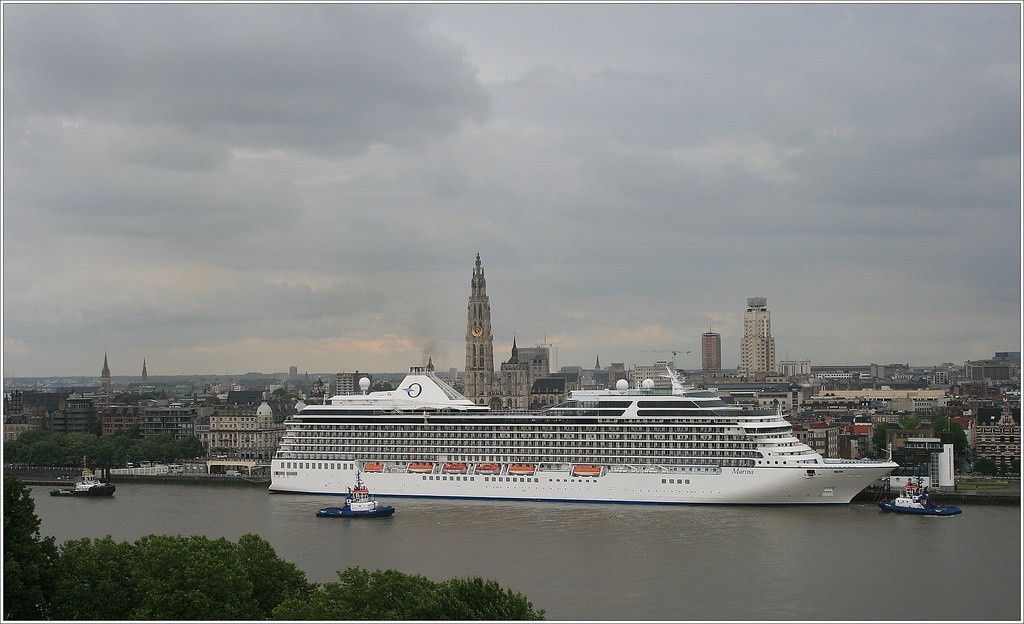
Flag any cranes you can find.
[643,350,691,362]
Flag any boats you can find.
[573,468,600,476]
[409,466,433,473]
[316,470,395,517]
[508,466,534,475]
[365,464,383,472]
[475,465,500,474]
[48,456,116,497]
[878,476,962,516]
[443,464,467,473]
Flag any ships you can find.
[269,356,899,505]
